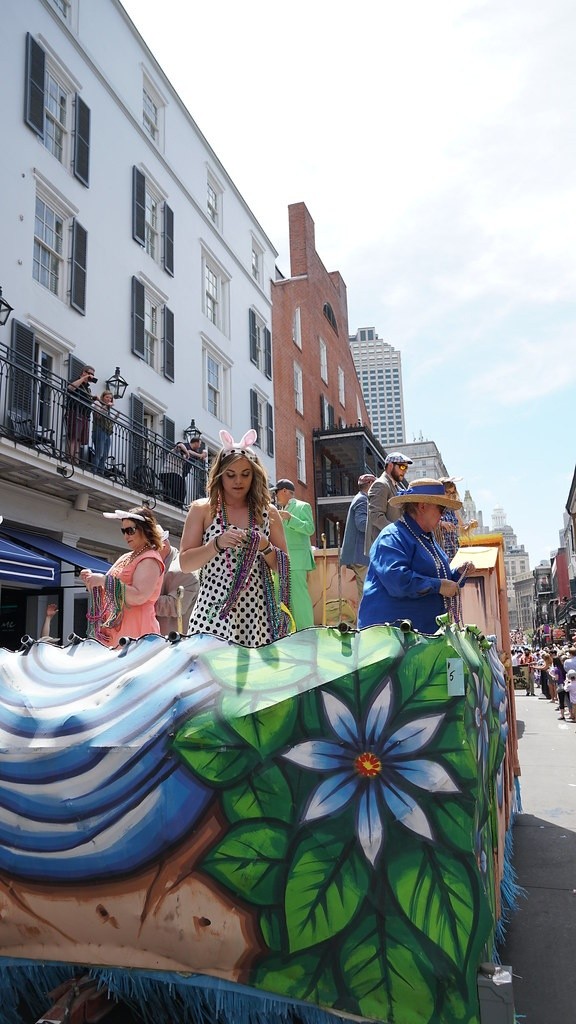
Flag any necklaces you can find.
[114,545,148,576]
[206,498,261,622]
[261,546,292,640]
[403,511,468,628]
[86,575,126,636]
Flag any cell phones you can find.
[88,377,98,383]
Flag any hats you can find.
[388,478,462,511]
[358,474,377,485]
[268,479,295,493]
[152,525,170,543]
[384,452,414,466]
[567,670,576,679]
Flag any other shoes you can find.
[557,717,565,721]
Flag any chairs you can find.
[86,446,127,485]
[134,465,172,501]
[7,409,60,454]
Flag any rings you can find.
[449,524,450,526]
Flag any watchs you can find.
[260,542,272,556]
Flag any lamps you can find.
[0,285,16,325]
[73,493,90,511]
[106,366,129,399]
[184,419,202,444]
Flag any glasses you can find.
[443,490,456,497]
[120,524,140,535]
[84,371,94,377]
[395,463,408,471]
[427,503,447,516]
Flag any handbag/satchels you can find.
[555,684,565,692]
[101,416,114,435]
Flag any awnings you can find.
[0,537,62,587]
[0,526,112,574]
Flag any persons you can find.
[510,626,576,724]
[179,443,290,647]
[40,603,58,637]
[340,474,378,605]
[364,452,414,556]
[155,525,199,637]
[65,366,100,465]
[358,478,476,636]
[92,390,121,475]
[79,507,165,644]
[439,476,478,559]
[270,479,316,631]
[160,437,208,505]
